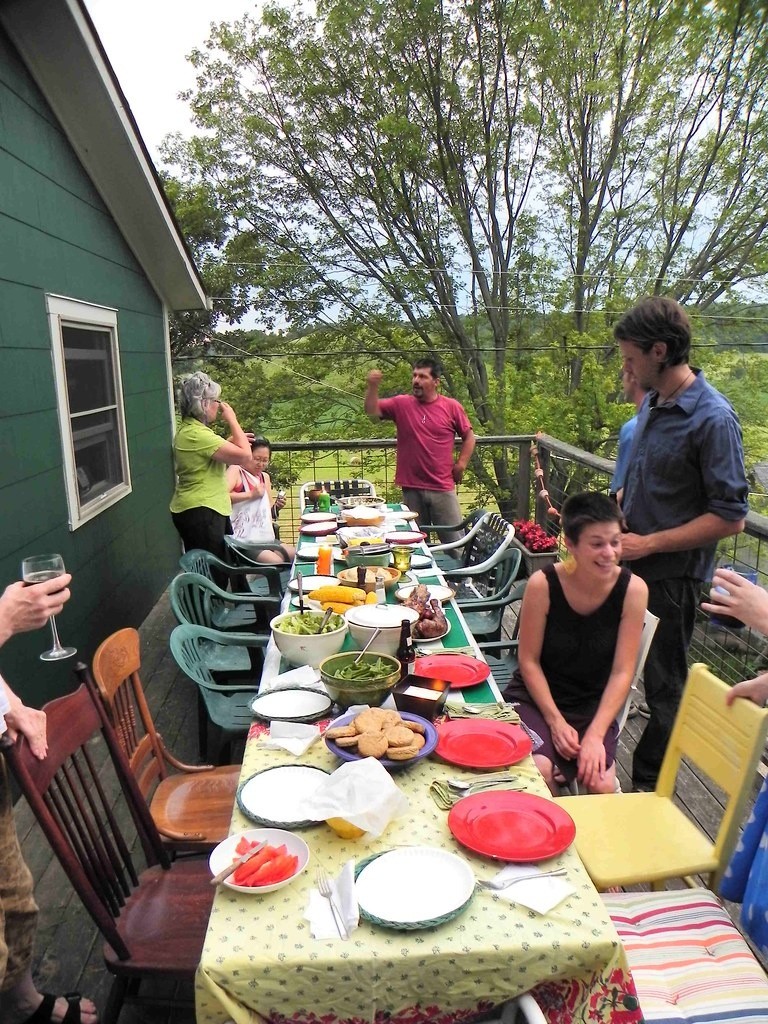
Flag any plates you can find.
[430,717,533,767]
[447,789,576,864]
[232,511,488,827]
[325,710,439,767]
[355,843,476,922]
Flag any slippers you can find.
[19,992,98,1024]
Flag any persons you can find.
[364,358,476,560]
[614,298,749,793]
[225,437,297,583]
[700,567,768,954]
[0,572,101,1024]
[503,491,649,893]
[610,364,651,509]
[168,370,256,626]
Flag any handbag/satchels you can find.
[227,465,276,545]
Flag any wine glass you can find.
[22,554,77,661]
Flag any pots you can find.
[344,541,391,568]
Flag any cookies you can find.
[326,707,425,761]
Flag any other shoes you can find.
[639,702,653,720]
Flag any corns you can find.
[308,586,378,614]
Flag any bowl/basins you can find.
[334,496,386,512]
[270,609,348,670]
[209,827,310,894]
[319,651,402,709]
[346,604,419,658]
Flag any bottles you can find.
[397,620,415,683]
[374,576,387,604]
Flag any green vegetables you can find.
[274,612,344,635]
[332,657,395,679]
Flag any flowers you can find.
[511,515,557,551]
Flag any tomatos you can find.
[232,837,298,887]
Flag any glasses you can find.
[255,435,269,444]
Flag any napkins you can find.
[415,646,477,660]
[411,567,448,579]
[482,862,576,913]
[303,756,399,842]
[443,703,521,725]
[269,664,322,692]
[300,856,361,939]
[259,719,321,755]
[429,769,528,809]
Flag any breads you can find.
[346,566,393,582]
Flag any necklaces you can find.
[659,370,693,407]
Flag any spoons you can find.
[447,775,516,789]
[463,702,520,714]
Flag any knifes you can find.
[210,838,268,886]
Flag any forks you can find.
[314,868,351,941]
[479,869,568,889]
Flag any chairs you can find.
[596,778,768,1024]
[166,522,293,766]
[300,480,376,515]
[549,661,767,892]
[418,508,660,796]
[0,628,243,1024]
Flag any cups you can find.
[710,565,757,629]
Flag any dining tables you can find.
[196,497,644,1024]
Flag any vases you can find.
[509,538,557,576]
